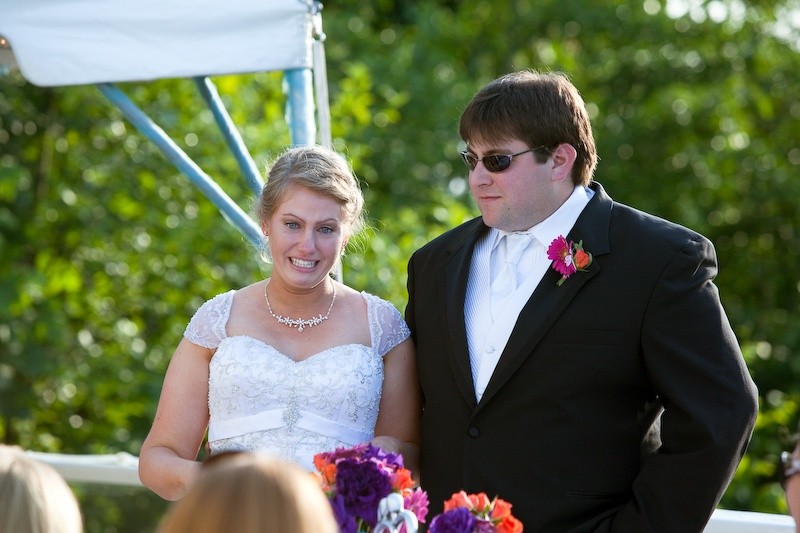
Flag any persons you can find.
[139,145,422,501]
[0,444,83,533]
[405,72,759,533]
[781,430,800,533]
[158,451,342,533]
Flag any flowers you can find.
[547,235,592,280]
[314,439,521,533]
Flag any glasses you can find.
[458,140,575,173]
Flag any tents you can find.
[0,0,331,265]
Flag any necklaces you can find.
[265,280,336,332]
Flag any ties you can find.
[484,232,535,351]
